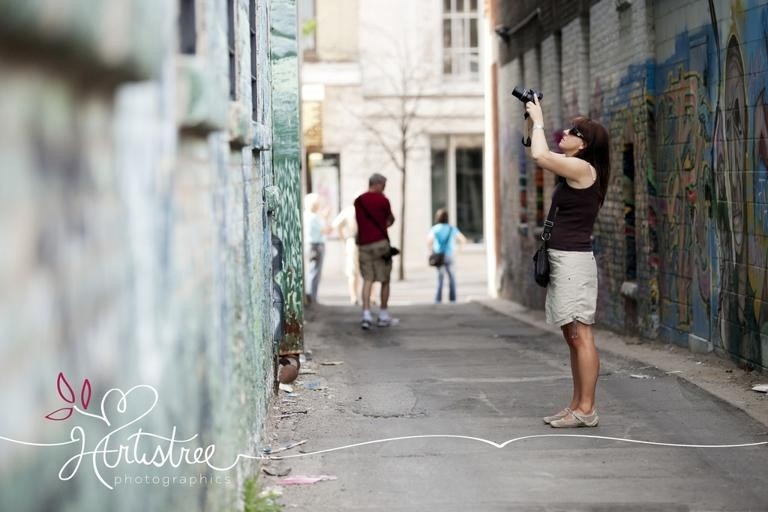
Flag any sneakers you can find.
[549,411,598,428]
[375,317,398,327]
[543,408,572,424]
[360,320,371,330]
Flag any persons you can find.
[525,92,609,431]
[425,208,468,304]
[353,172,400,329]
[332,203,376,306]
[302,191,332,304]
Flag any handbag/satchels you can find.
[532,243,549,288]
[429,254,444,266]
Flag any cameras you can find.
[512,86,543,120]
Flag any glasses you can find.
[569,127,584,139]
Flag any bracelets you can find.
[531,124,543,130]
